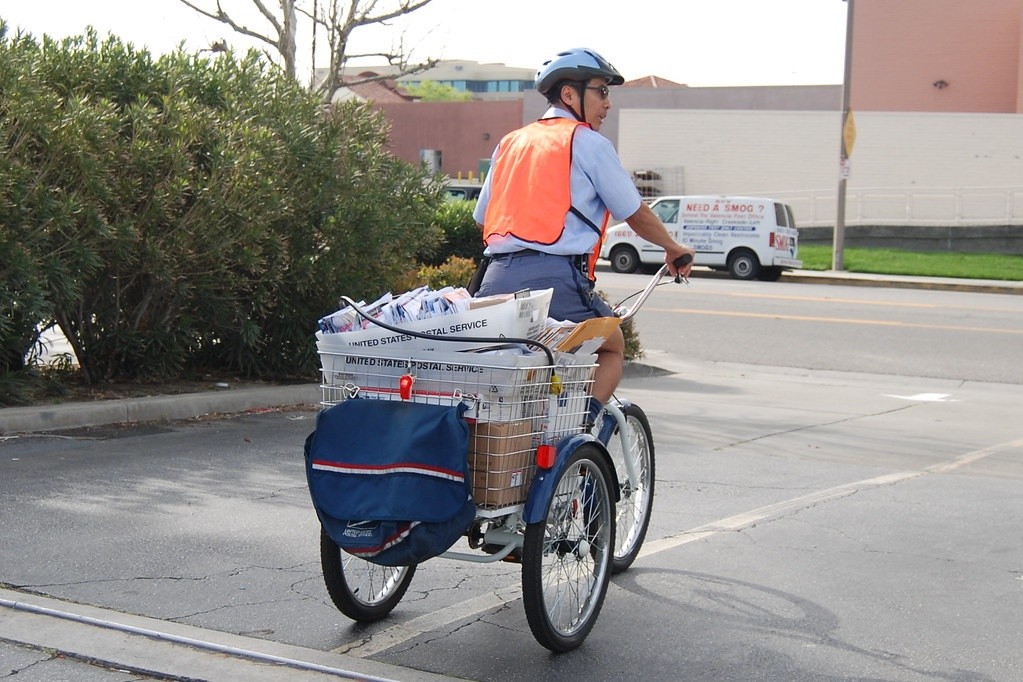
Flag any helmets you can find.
[535,46,624,99]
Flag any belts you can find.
[489,248,572,262]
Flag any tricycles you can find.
[316,253,694,653]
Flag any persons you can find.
[473,49,695,434]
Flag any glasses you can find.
[569,84,609,99]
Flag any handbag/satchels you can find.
[303,394,477,568]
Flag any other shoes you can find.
[578,422,595,475]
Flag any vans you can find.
[599,194,803,281]
